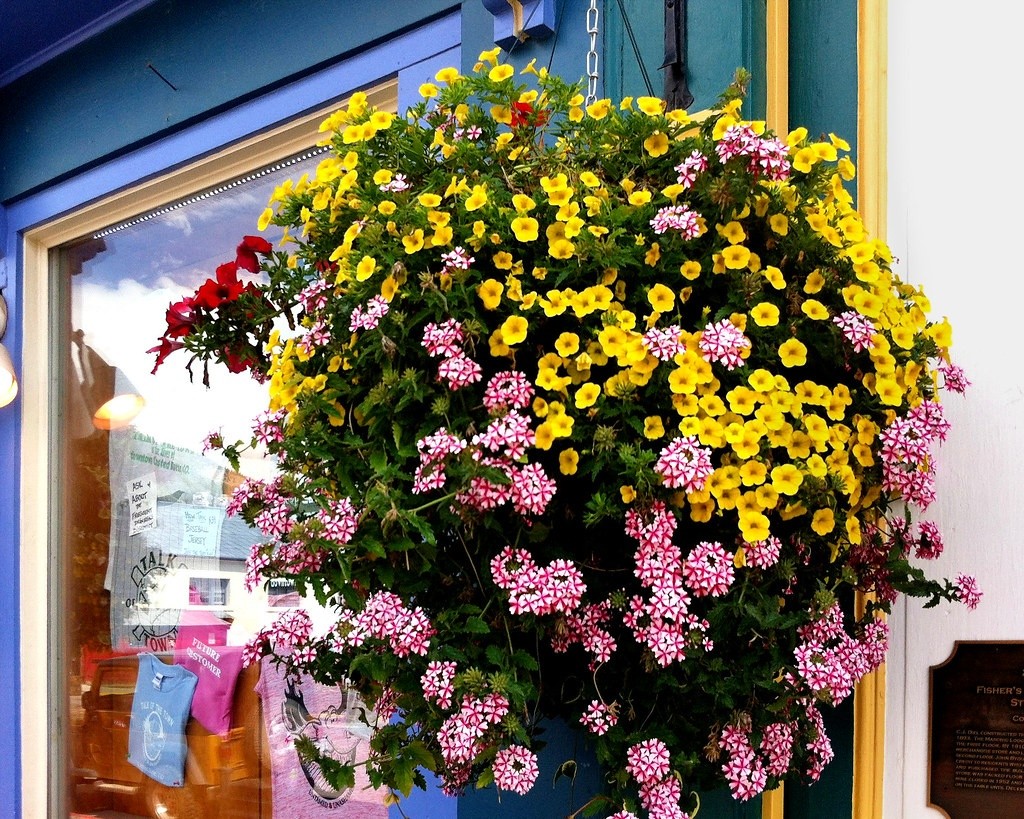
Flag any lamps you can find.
[0,293,20,409]
[73,335,145,431]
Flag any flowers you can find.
[142,44,986,819]
